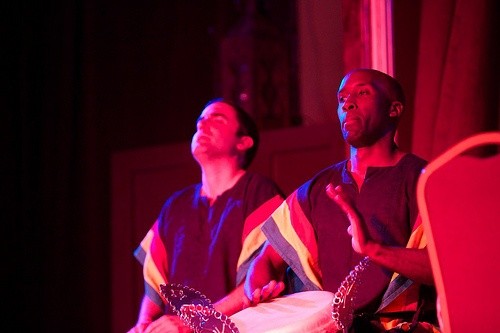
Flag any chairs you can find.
[415,131,500,333]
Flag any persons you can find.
[244,68,437,333]
[127,97,289,333]
